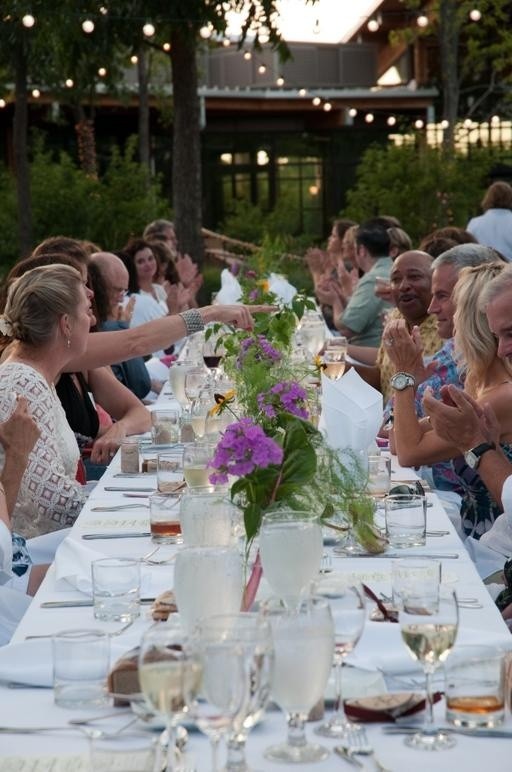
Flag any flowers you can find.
[218,231,332,605]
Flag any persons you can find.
[305,181,512,631]
[1,219,281,641]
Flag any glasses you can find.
[113,287,130,297]
[384,480,425,504]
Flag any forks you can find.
[26,621,135,639]
[0,717,143,743]
[90,496,184,512]
[344,726,391,772]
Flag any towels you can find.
[51,536,120,593]
[314,365,385,450]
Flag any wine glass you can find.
[400,589,458,752]
[258,510,325,609]
[258,593,334,764]
[142,620,203,772]
[309,582,366,739]
[169,360,202,419]
[185,629,252,770]
[199,611,271,771]
[179,485,236,548]
[173,546,245,644]
[201,340,225,387]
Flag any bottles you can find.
[121,440,140,474]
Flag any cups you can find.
[298,312,349,381]
[385,493,428,548]
[53,629,112,711]
[446,642,509,729]
[92,559,139,620]
[392,560,441,616]
[145,372,240,495]
[368,456,392,496]
